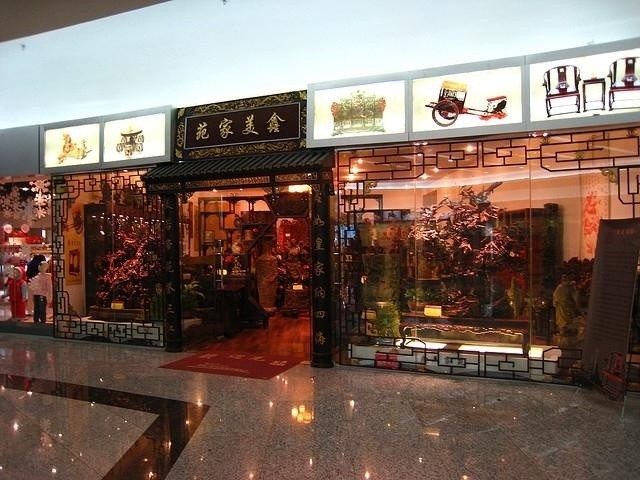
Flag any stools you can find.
[116,135,144,157]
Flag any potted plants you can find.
[181,279,205,319]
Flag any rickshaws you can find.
[65,212,84,235]
[425,80,507,127]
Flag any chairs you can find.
[606,56,640,111]
[541,64,580,118]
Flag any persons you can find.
[552,271,593,337]
[30,262,53,324]
[4,267,28,321]
[629,264,640,344]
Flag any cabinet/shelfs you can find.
[83,202,194,317]
[196,194,277,274]
[0,243,53,305]
[335,202,560,350]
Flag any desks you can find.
[204,283,247,329]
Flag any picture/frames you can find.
[175,89,307,160]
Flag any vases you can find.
[255,240,279,309]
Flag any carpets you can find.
[158,352,305,380]
